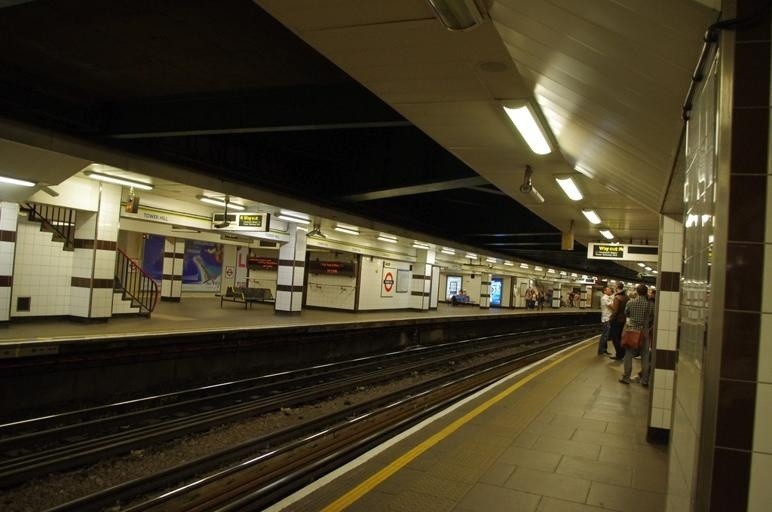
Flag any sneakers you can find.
[597,351,649,388]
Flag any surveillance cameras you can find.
[215,223,228,228]
[316,231,326,239]
[520,184,544,204]
[306,230,316,237]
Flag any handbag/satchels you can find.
[620,328,644,349]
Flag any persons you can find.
[560,290,580,308]
[596,282,656,386]
[524,287,545,312]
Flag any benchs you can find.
[214,285,276,311]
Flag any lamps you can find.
[429,0,484,34]
[273,211,312,225]
[499,96,615,241]
[195,193,248,212]
[83,170,153,192]
[331,222,430,252]
